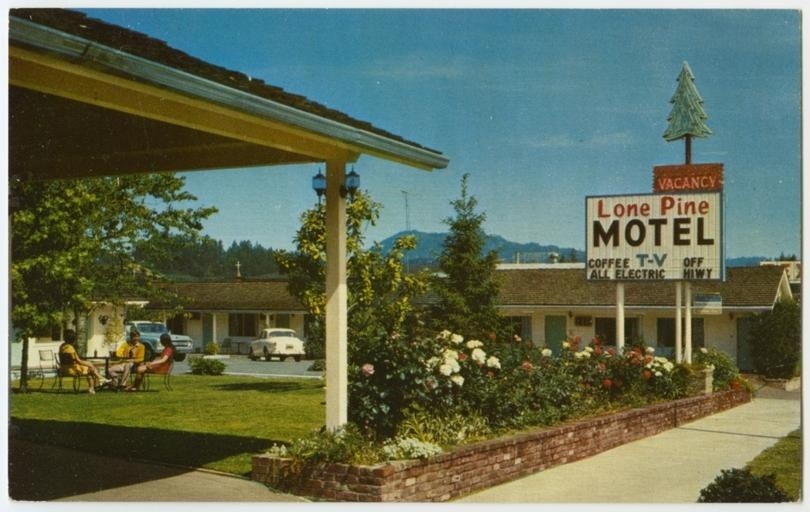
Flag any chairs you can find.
[32,343,178,395]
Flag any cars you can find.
[248,328,306,362]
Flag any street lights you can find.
[399,189,412,273]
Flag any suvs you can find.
[126,320,194,360]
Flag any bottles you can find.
[129,350,133,357]
[93,350,97,357]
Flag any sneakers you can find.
[99,378,113,386]
[88,388,97,395]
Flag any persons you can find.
[59,328,113,393]
[125,334,175,391]
[110,330,145,388]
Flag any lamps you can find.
[339,162,362,205]
[312,165,327,208]
